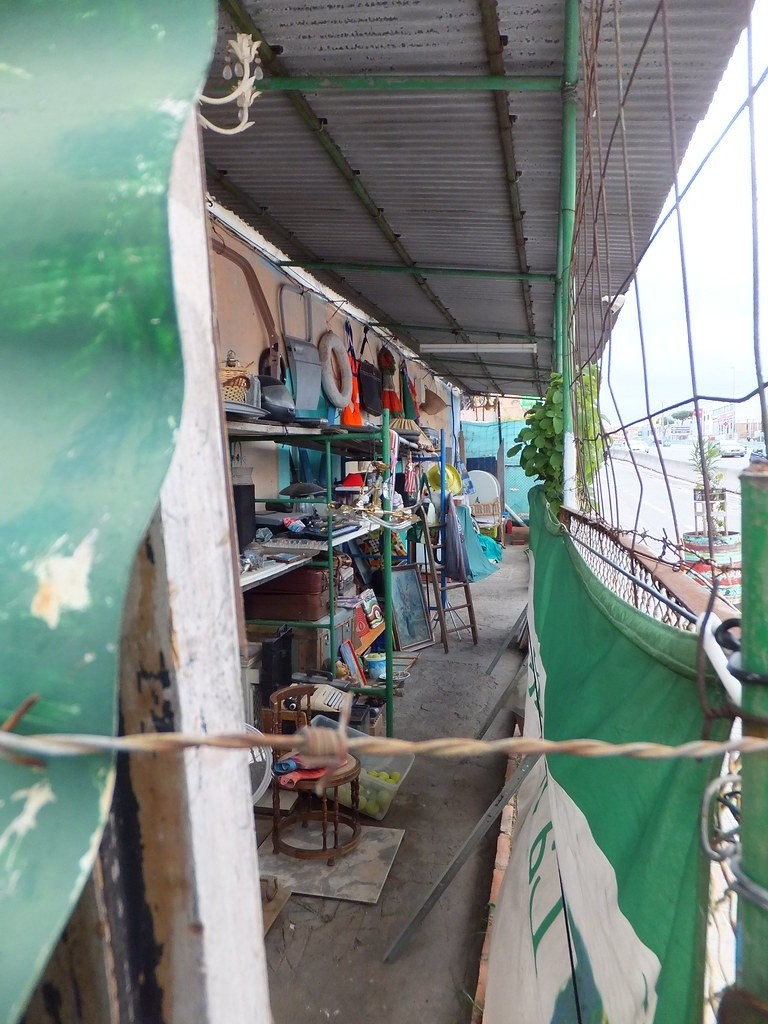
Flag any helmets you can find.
[253,375,296,423]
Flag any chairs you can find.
[270,685,362,867]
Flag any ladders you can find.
[418,490,480,656]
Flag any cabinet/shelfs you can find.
[227,409,445,738]
[244,607,355,674]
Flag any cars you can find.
[747,441,768,464]
[717,439,746,458]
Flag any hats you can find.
[428,463,462,496]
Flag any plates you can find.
[380,671,411,682]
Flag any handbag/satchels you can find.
[340,319,384,427]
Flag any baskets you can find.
[422,385,451,415]
[218,367,250,403]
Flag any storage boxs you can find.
[291,715,415,821]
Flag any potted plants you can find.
[684,434,742,605]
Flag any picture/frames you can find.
[374,564,436,651]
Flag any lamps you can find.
[420,343,537,354]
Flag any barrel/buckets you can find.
[683,530,742,604]
[365,653,386,679]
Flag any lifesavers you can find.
[318,332,352,406]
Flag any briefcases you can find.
[242,547,356,709]
[420,427,439,449]
